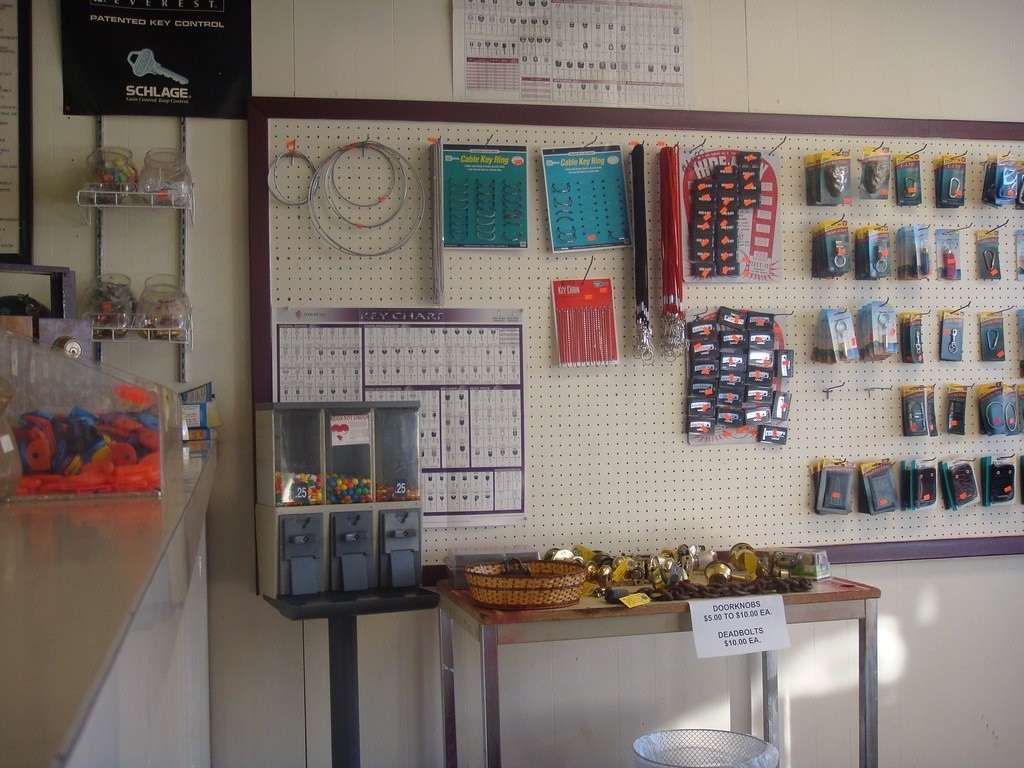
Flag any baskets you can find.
[464,558,588,610]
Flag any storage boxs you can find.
[0,327,184,498]
[256,400,422,508]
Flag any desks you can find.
[264,588,440,768]
[437,575,882,768]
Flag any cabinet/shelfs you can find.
[78,117,196,381]
